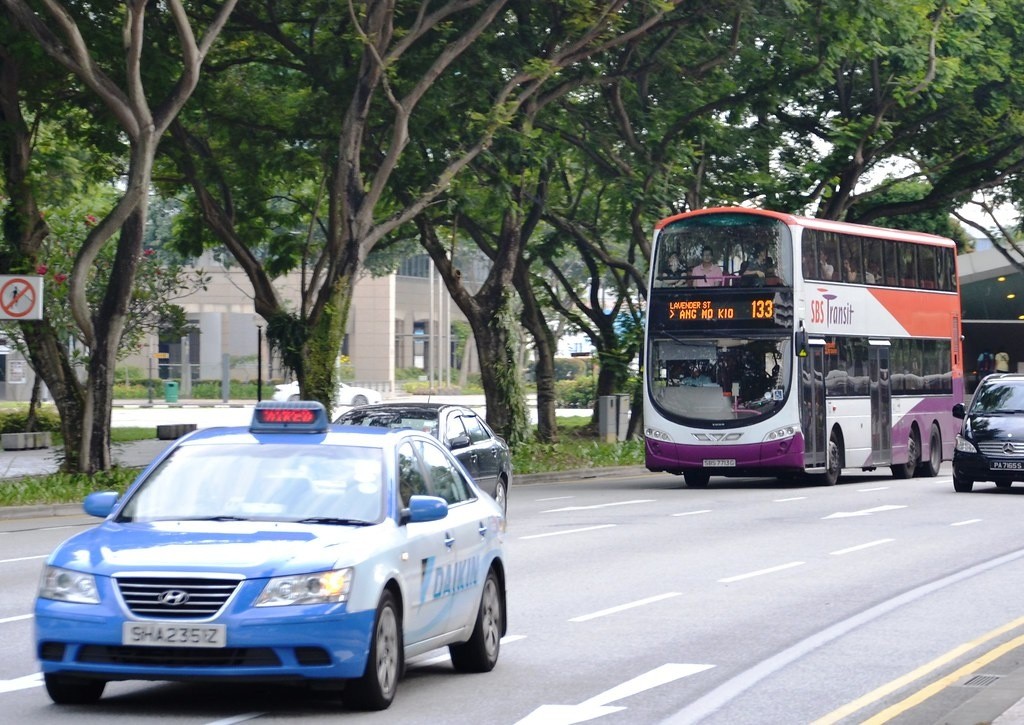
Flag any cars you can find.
[949,373,1023,494]
[272,379,381,407]
[33,398,509,712]
[331,406,511,512]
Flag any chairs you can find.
[687,269,935,287]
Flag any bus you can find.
[639,207,967,486]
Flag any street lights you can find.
[254,315,267,403]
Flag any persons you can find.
[818,247,834,281]
[994,352,1010,373]
[977,348,994,382]
[679,366,712,387]
[743,250,775,287]
[844,258,856,283]
[864,257,875,283]
[661,251,687,287]
[692,247,724,287]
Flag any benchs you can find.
[250,475,382,523]
[157,424,196,441]
[1,431,51,451]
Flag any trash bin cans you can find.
[164,381,178,404]
